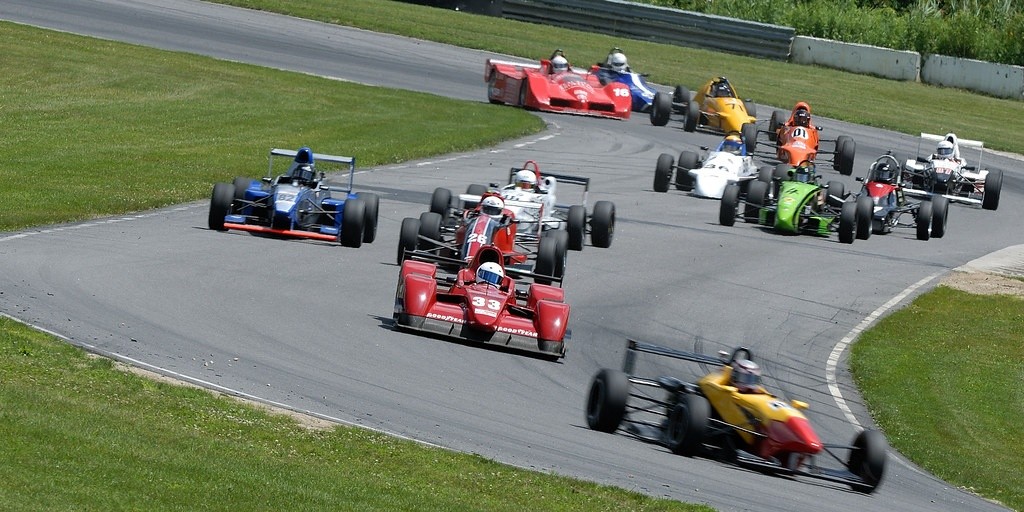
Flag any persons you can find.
[716,83,732,97]
[514,169,537,193]
[291,163,316,190]
[551,55,568,74]
[936,141,960,165]
[795,166,811,183]
[722,135,743,156]
[875,163,897,184]
[610,53,629,75]
[469,262,504,290]
[729,359,762,394]
[793,109,811,128]
[475,196,505,223]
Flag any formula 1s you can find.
[587,332,888,495]
[485,46,787,135]
[208,147,380,249]
[651,100,1004,244]
[395,163,616,365]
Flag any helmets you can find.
[876,163,893,182]
[515,170,536,192]
[729,359,762,389]
[794,166,811,184]
[480,196,504,219]
[722,136,742,154]
[937,141,954,158]
[292,164,316,185]
[476,262,504,289]
[552,56,568,74]
[716,83,730,96]
[793,110,810,126]
[611,53,627,71]
